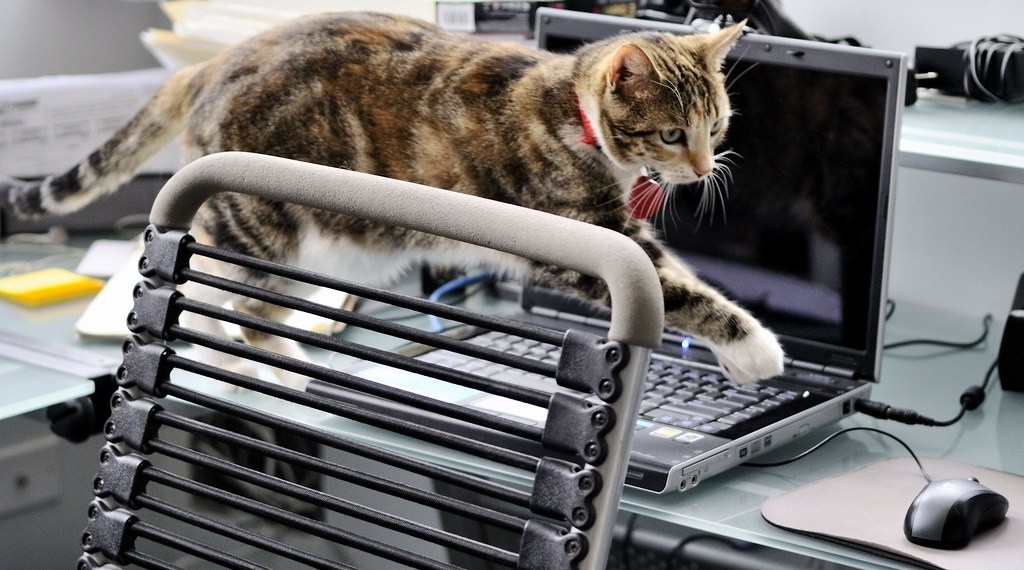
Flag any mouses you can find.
[903,478,1010,550]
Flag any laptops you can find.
[307,6,908,493]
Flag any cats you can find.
[0,10,785,384]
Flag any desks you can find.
[0,233,1024,570]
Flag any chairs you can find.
[76,150,664,570]
